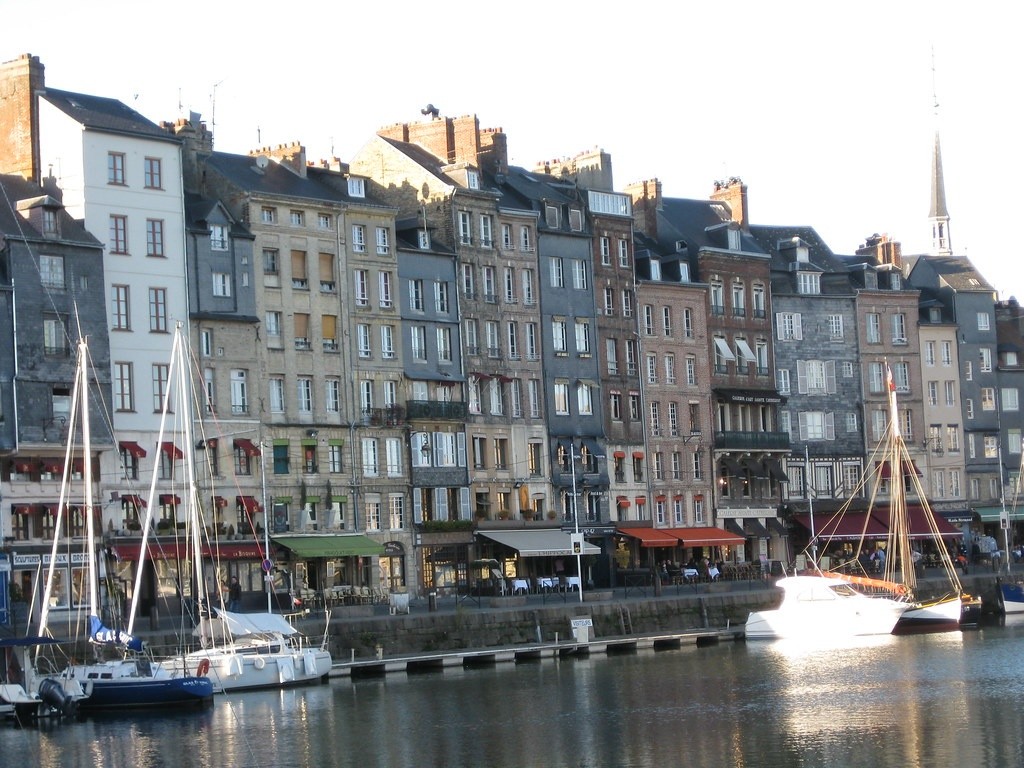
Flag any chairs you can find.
[664,563,767,585]
[506,574,589,596]
[291,585,387,610]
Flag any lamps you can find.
[42,415,69,446]
[683,435,706,457]
[921,436,945,458]
[407,431,433,457]
[195,440,208,449]
[307,428,319,437]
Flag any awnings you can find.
[12,459,99,473]
[120,438,261,458]
[792,500,964,542]
[10,503,101,517]
[477,529,601,557]
[715,337,758,362]
[617,496,631,508]
[580,378,601,387]
[122,495,262,514]
[875,460,924,480]
[617,527,746,548]
[271,535,387,558]
[560,437,606,458]
[720,458,792,482]
[972,506,1024,522]
[713,388,787,406]
[724,518,789,538]
[404,370,514,383]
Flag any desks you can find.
[537,578,553,593]
[708,568,720,582]
[565,576,580,593]
[512,580,528,595]
[551,577,559,592]
[683,568,698,583]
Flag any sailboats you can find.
[0,290,333,725]
[742,355,985,642]
[983,430,1024,616]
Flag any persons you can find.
[216,576,244,613]
[1003,541,1024,558]
[659,558,725,583]
[835,543,970,579]
[554,556,566,578]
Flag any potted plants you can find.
[498,508,509,520]
[129,522,142,536]
[157,521,171,535]
[243,524,254,540]
[255,522,264,540]
[212,522,227,541]
[228,524,236,541]
[549,510,556,521]
[522,509,534,520]
[476,508,488,520]
[177,521,185,536]
